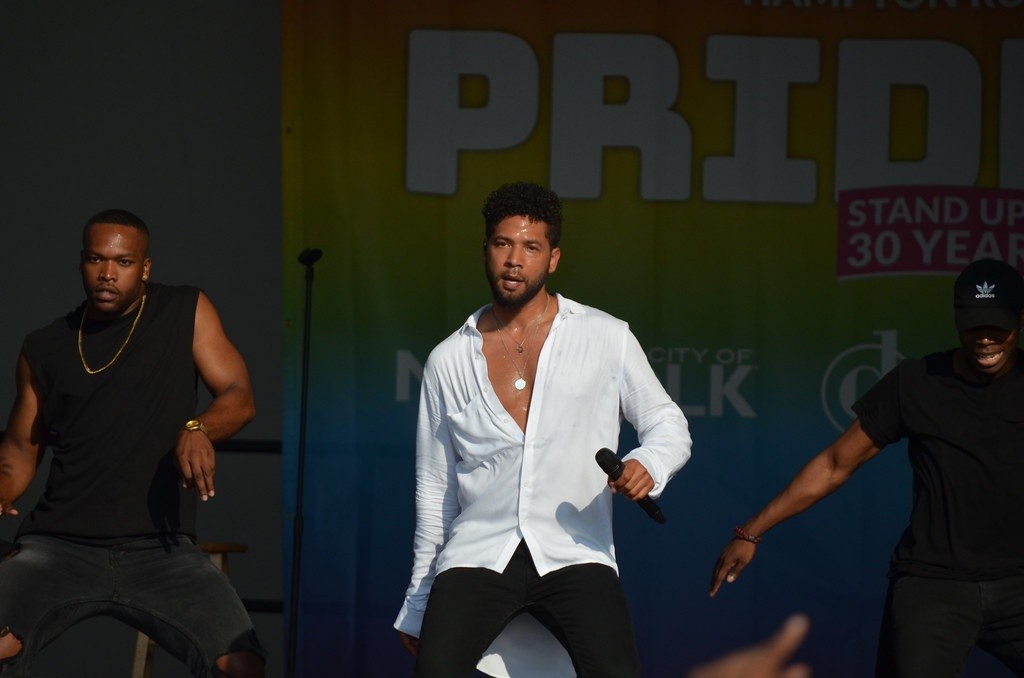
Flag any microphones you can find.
[595,448,666,524]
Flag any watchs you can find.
[181,419,208,436]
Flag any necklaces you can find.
[487,290,552,389]
[78,288,148,375]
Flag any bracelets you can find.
[734,525,763,543]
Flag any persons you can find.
[0,202,268,678]
[389,177,689,678]
[694,612,818,677]
[708,254,1024,678]
